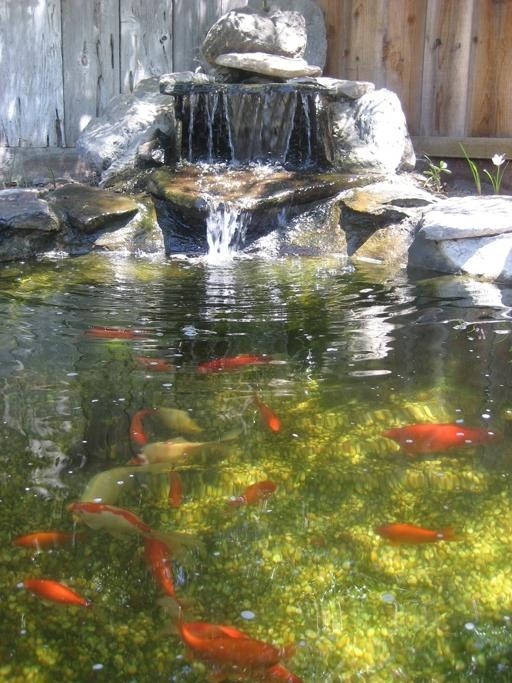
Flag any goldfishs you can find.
[11,352,285,613]
